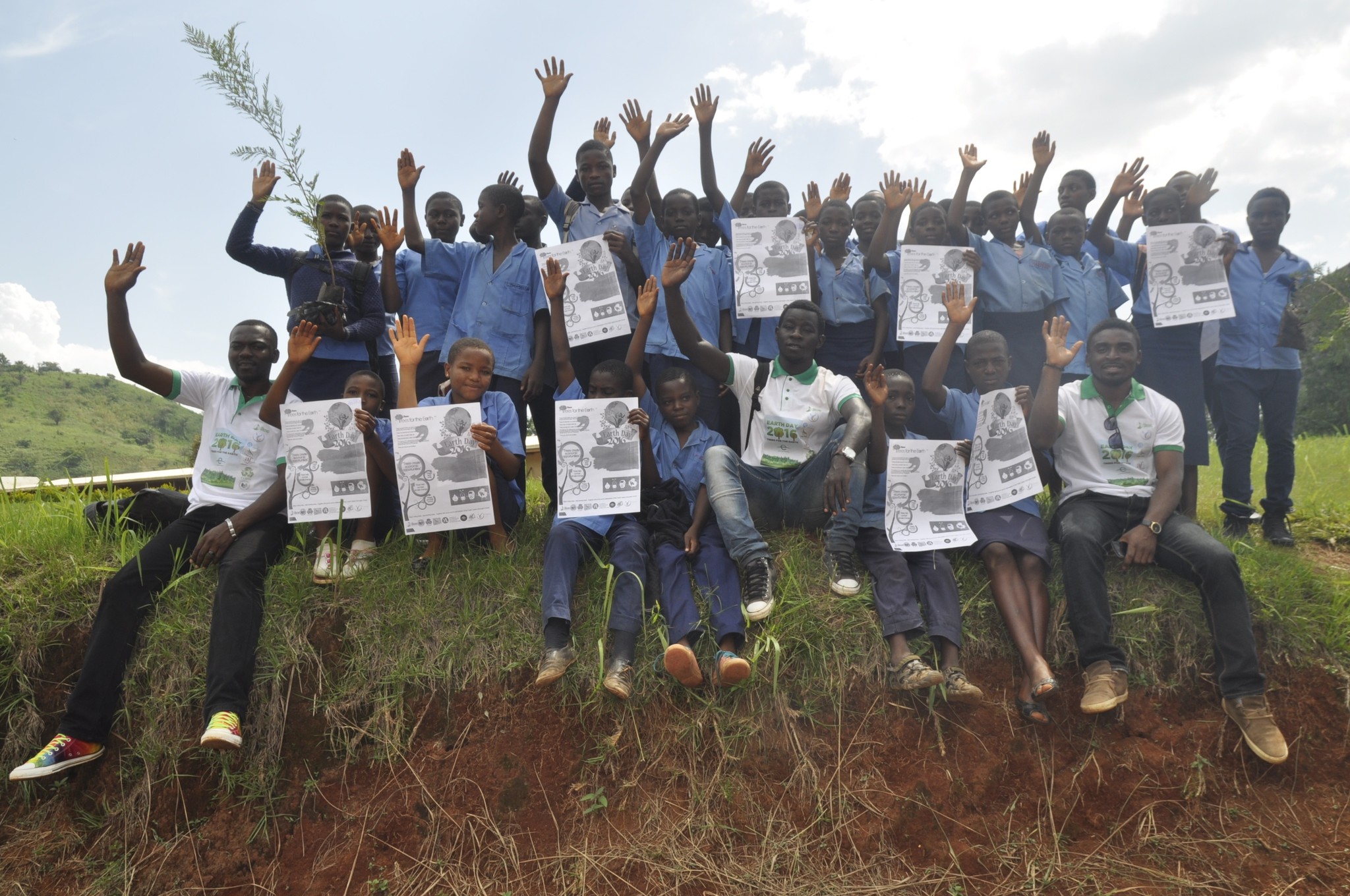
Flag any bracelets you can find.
[1043,362,1065,371]
[225,517,238,541]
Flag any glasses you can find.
[1104,414,1126,452]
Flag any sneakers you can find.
[713,650,751,685]
[1222,692,1289,764]
[743,557,774,621]
[1258,512,1296,545]
[822,545,861,597]
[602,657,646,700]
[1222,514,1256,546]
[653,644,703,687]
[535,634,582,685]
[9,732,105,782]
[1080,659,1128,714]
[202,712,243,750]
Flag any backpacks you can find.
[84,488,191,539]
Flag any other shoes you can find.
[1031,676,1060,703]
[342,539,382,580]
[936,667,983,702]
[886,652,944,690]
[313,542,341,584]
[1016,696,1052,726]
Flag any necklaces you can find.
[242,391,253,398]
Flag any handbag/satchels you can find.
[1272,276,1307,351]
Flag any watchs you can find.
[1140,519,1162,534]
[833,446,857,464]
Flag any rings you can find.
[208,551,215,555]
[128,259,133,262]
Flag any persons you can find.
[258,320,392,584]
[1181,167,1316,548]
[388,314,528,575]
[534,256,657,697]
[83,56,1240,525]
[1027,315,1289,763]
[923,280,1061,724]
[9,241,305,781]
[624,275,752,692]
[857,363,984,704]
[662,237,871,620]
